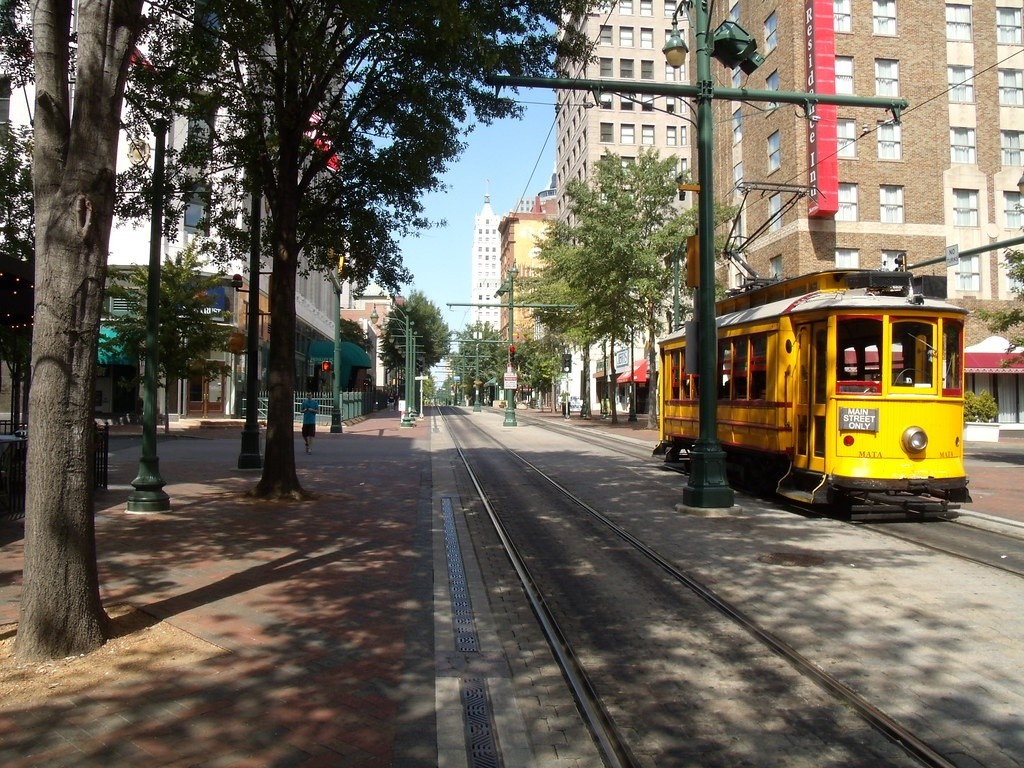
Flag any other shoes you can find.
[305,446,312,454]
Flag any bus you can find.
[653,268,971,522]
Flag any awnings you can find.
[616,358,647,382]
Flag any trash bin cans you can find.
[561,396,571,419]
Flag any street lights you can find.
[502,258,519,425]
[562,354,572,373]
[474,319,482,413]
[370,302,418,427]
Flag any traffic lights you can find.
[322,361,332,373]
[510,346,516,363]
[452,384,455,391]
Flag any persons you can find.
[300,389,319,455]
[387,393,395,411]
